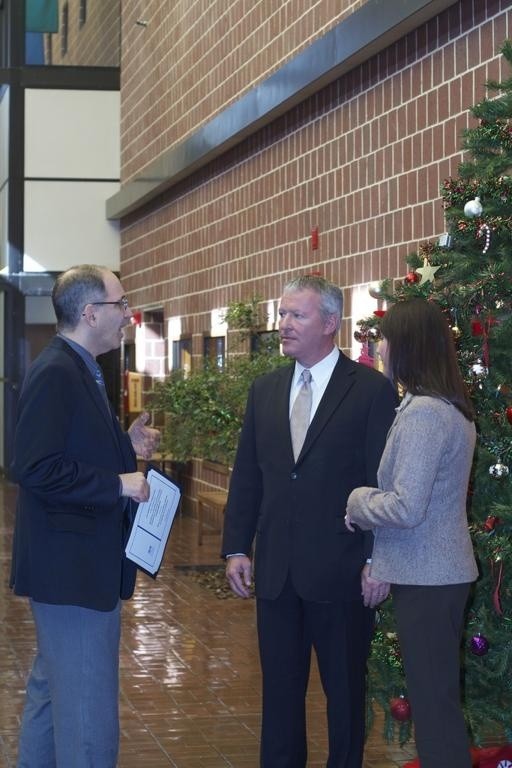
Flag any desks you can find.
[135,451,194,519]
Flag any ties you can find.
[289,370,312,464]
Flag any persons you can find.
[8,265,161,768]
[221,275,399,768]
[345,299,479,768]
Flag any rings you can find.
[379,593,384,596]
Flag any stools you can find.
[197,490,229,556]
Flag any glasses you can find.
[82,296,128,316]
